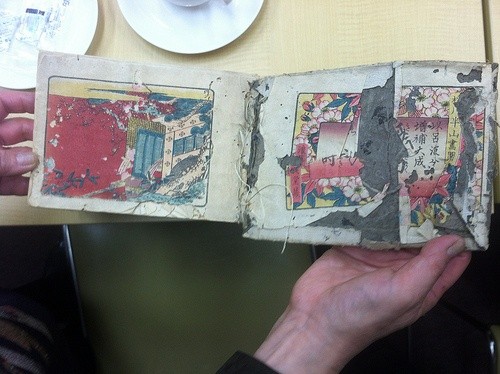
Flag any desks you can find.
[0,0,499,236]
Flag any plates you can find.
[0,1,98,90]
[118,0,265,54]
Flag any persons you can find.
[0,88,476,374]
